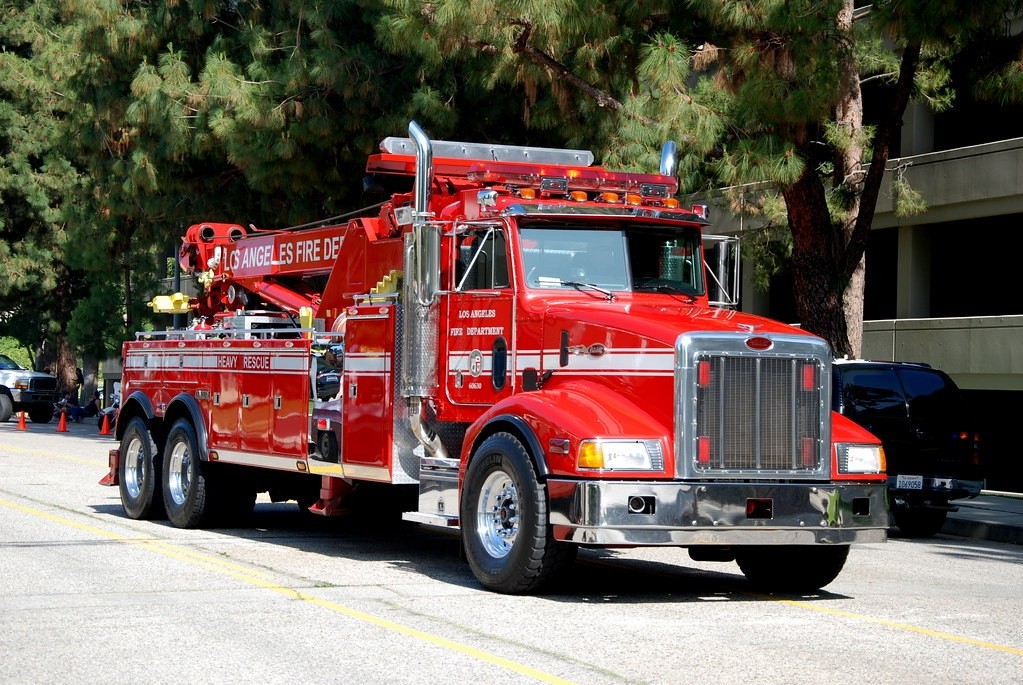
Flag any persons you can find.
[67,391,101,423]
[53,368,84,417]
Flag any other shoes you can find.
[69,419,80,423]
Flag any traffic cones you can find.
[55,412,70,432]
[15,410,29,431]
[98,414,112,435]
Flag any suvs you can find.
[832,354,986,538]
[0,354,57,423]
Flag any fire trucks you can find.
[98,121,890,595]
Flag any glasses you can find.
[92,394,95,395]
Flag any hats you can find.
[92,390,100,398]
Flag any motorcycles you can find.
[98,382,121,430]
